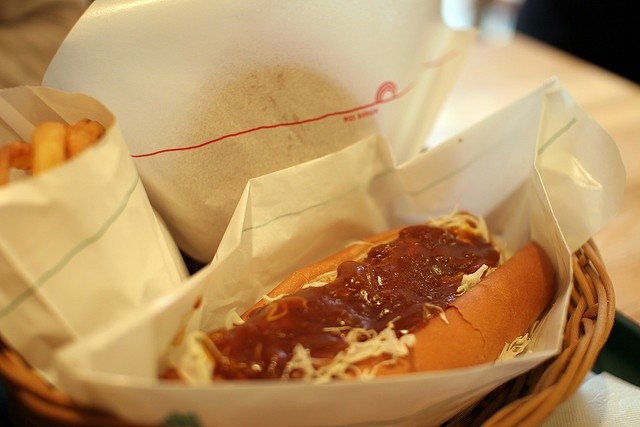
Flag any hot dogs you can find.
[155,212,560,388]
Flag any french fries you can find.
[0,117,108,187]
